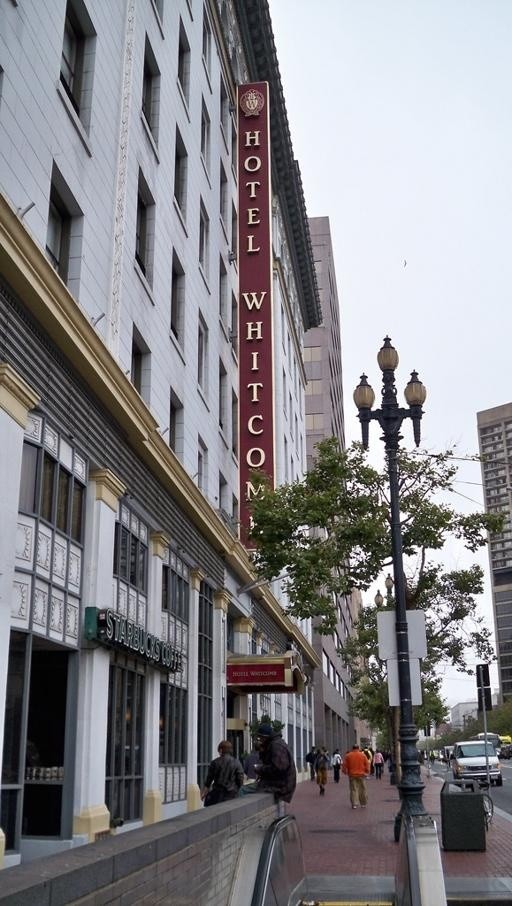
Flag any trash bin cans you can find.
[439,779,487,852]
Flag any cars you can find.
[420,746,453,763]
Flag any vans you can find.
[451,742,502,786]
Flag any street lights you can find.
[353,333,427,818]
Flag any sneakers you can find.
[320,786,325,795]
[353,805,366,808]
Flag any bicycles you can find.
[474,778,493,832]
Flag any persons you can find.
[306,746,343,783]
[245,744,265,784]
[360,746,390,779]
[313,746,330,795]
[501,745,512,760]
[418,748,435,766]
[201,741,244,806]
[239,725,297,803]
[342,744,369,808]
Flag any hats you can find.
[256,726,277,737]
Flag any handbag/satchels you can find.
[204,792,223,806]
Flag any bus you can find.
[469,732,503,756]
